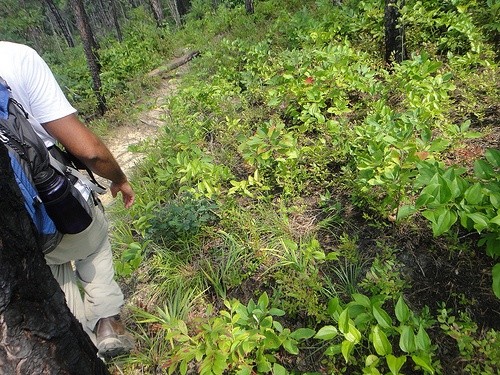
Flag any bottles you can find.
[34,167,93,234]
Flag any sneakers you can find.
[95,317,131,357]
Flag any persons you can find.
[0,37,137,363]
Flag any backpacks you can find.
[0,76,94,255]
